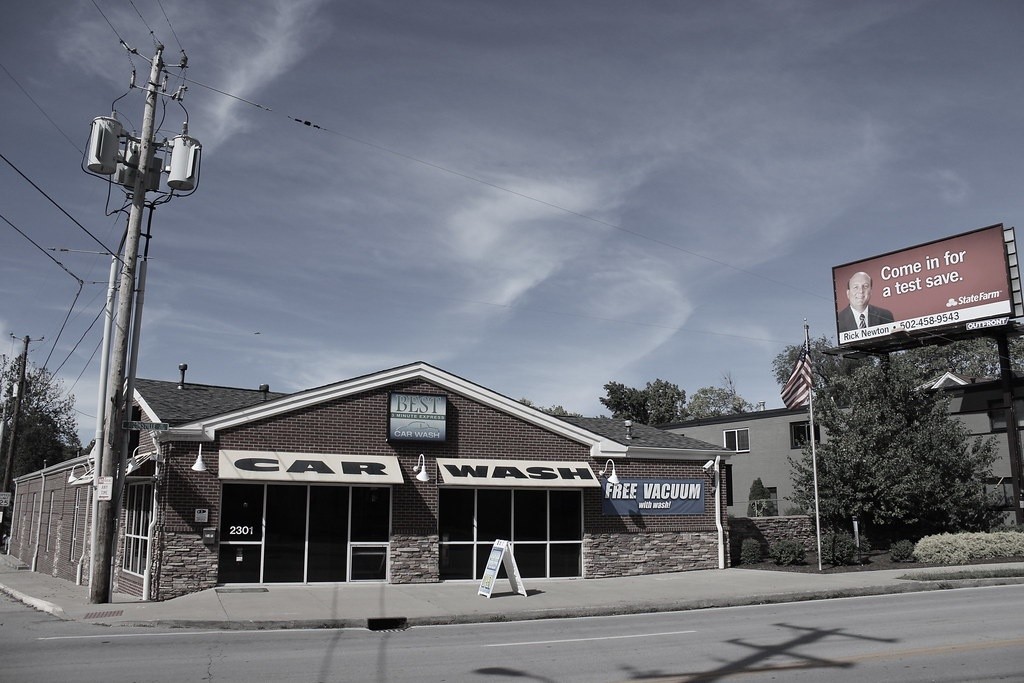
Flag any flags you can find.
[780,335,813,410]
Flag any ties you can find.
[859,314,866,328]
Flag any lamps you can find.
[67,464,87,484]
[598,458,620,484]
[412,453,430,482]
[190,444,207,472]
[126,444,156,475]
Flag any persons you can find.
[837,271,896,333]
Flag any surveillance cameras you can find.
[702,460,714,472]
